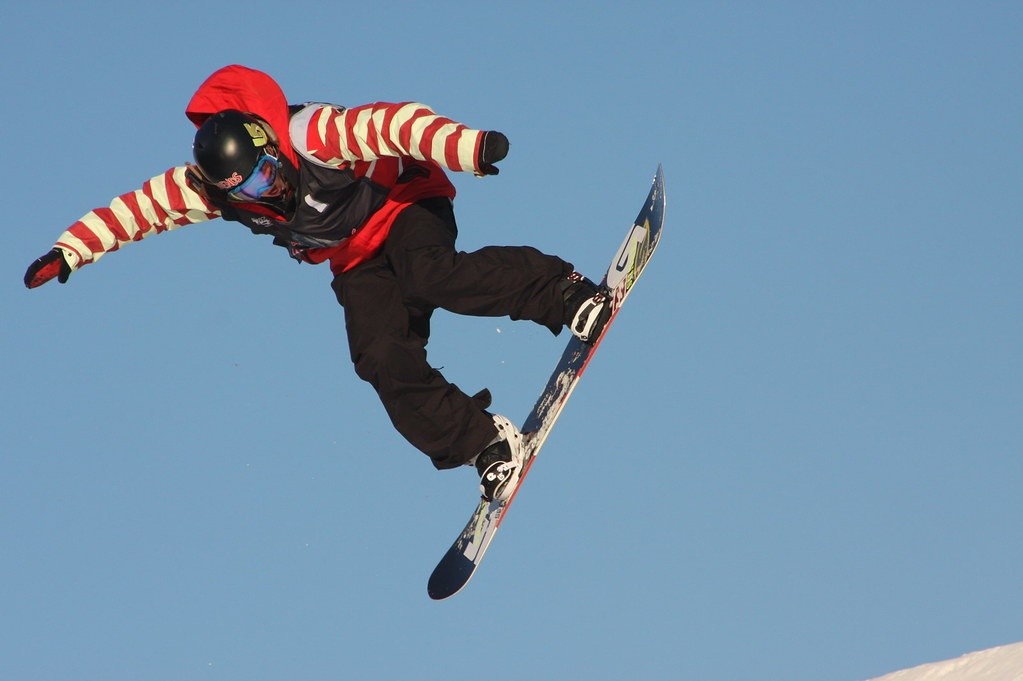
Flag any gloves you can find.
[25,245,71,289]
[475,129,509,176]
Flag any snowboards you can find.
[425,160,668,602]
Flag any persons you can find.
[19,62,612,502]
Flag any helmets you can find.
[191,108,280,201]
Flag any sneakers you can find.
[559,271,612,344]
[468,414,524,501]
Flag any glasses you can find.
[229,155,280,201]
